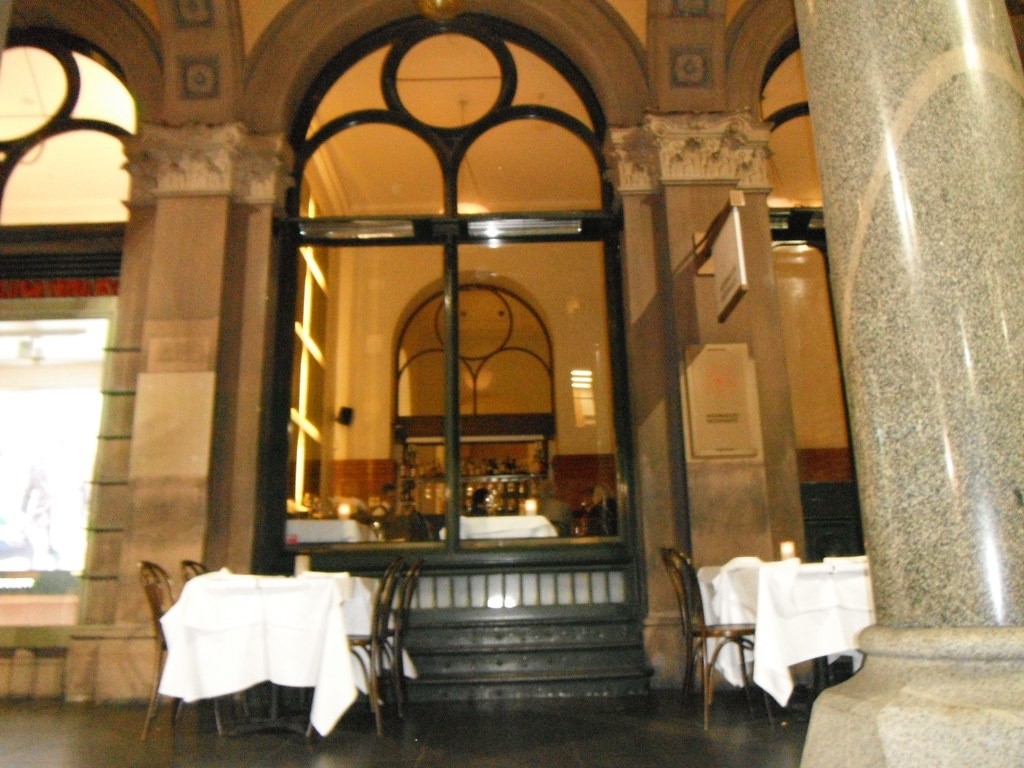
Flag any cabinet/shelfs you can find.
[398,438,551,517]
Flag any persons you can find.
[570,481,619,537]
[371,484,434,540]
[466,487,491,518]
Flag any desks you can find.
[284,514,379,545]
[438,514,559,540]
[695,554,876,732]
[175,568,383,736]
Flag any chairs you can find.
[657,544,778,735]
[301,553,428,741]
[135,558,251,738]
